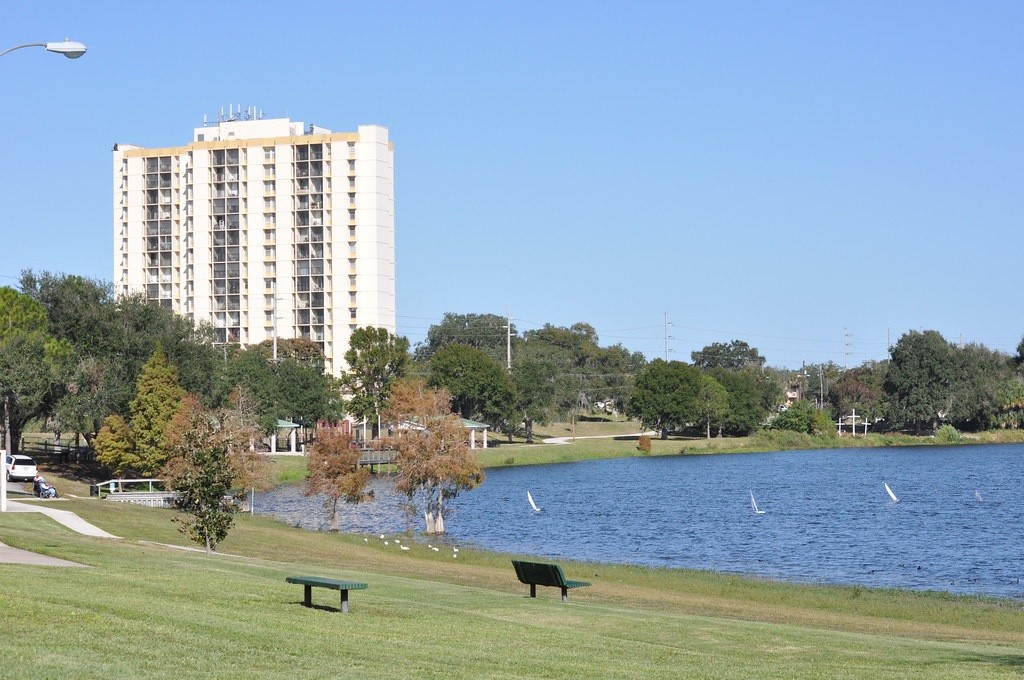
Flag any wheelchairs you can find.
[32,479,56,499]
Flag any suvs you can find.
[5,455,40,483]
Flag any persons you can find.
[34,465,57,498]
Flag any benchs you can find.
[286,575,369,613]
[511,560,591,602]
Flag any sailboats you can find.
[975,490,984,503]
[749,490,766,514]
[527,490,541,512]
[883,482,901,504]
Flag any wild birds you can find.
[428,544,440,552]
[384,541,389,546]
[379,534,384,538]
[364,538,368,543]
[400,544,410,551]
[453,546,459,558]
[395,539,400,544]
